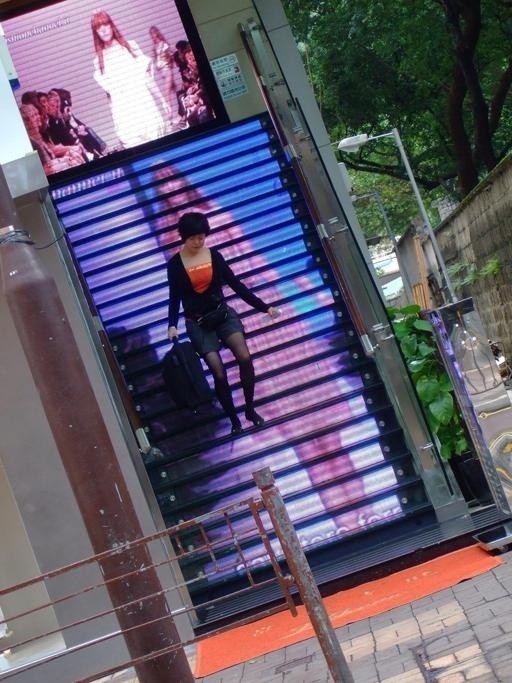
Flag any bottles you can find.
[260,308,284,324]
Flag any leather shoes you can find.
[232,417,241,434]
[246,412,264,428]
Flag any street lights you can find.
[338,127,458,304]
[349,191,418,306]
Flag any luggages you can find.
[161,336,213,411]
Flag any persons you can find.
[147,159,380,539]
[90,9,173,151]
[149,24,214,130]
[101,325,239,551]
[18,87,108,175]
[167,211,281,433]
